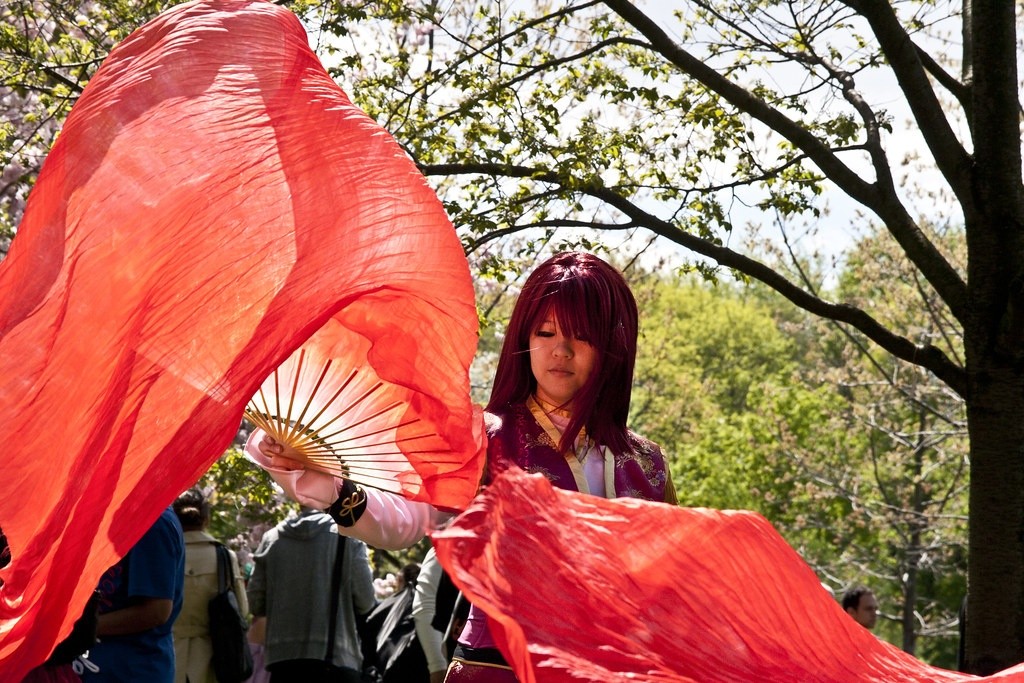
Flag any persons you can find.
[171,489,250,683]
[248,503,377,683]
[242,250,680,683]
[24,504,185,683]
[840,585,877,630]
[379,547,472,683]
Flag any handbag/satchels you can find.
[203,543,254,683]
